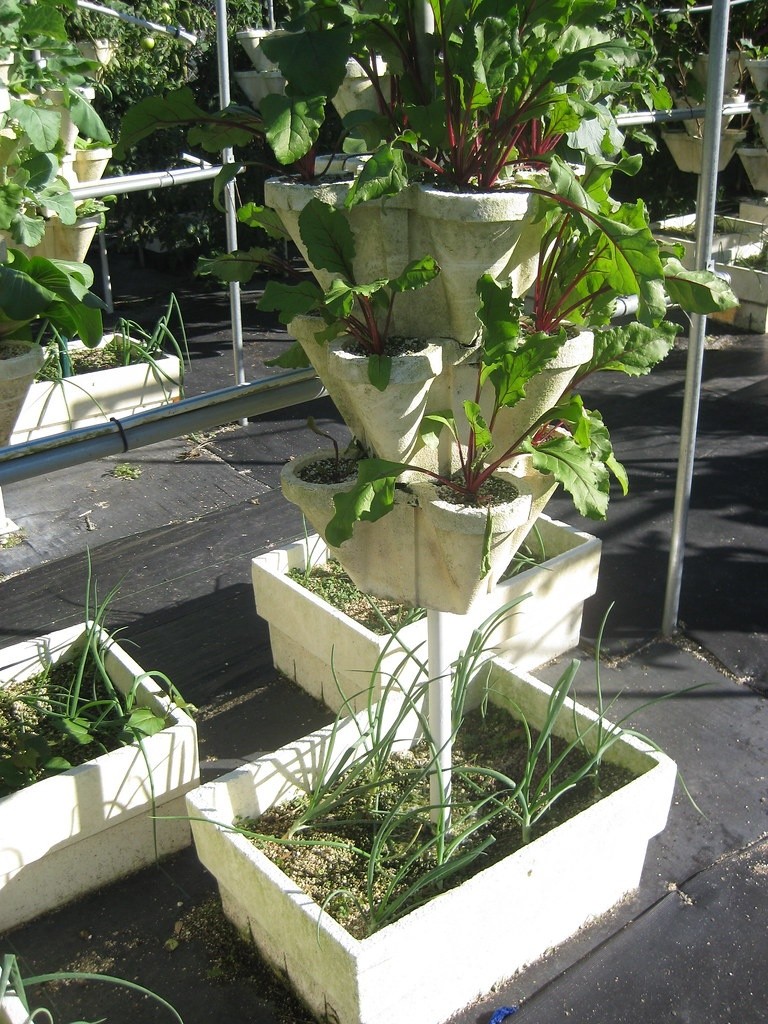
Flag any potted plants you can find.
[0,542,200,935]
[10,292,190,444]
[646,196,768,333]
[0,0,126,445]
[107,0,742,616]
[147,591,720,1024]
[655,0,768,194]
[251,512,603,717]
